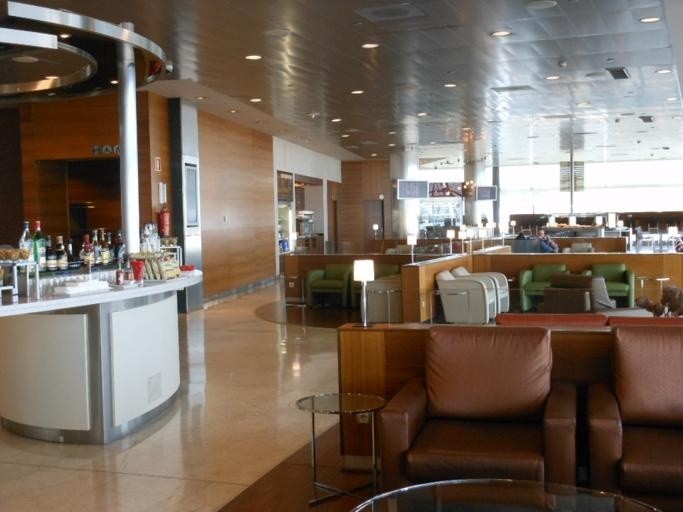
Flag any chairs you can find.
[588,324,682,509]
[378,325,579,484]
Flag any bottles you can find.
[140,222,160,256]
[81,227,123,265]
[16,220,73,273]
[115,256,132,286]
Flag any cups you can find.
[0,248,29,261]
[132,261,144,282]
[282,240,287,251]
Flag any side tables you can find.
[295,392,388,510]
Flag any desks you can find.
[634,275,671,304]
[276,270,307,308]
[360,273,402,323]
[429,290,472,324]
[504,272,519,314]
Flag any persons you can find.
[516,232,526,240]
[537,228,559,253]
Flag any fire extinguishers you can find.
[158,206,170,238]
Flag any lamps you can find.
[371,211,682,262]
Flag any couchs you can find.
[518,262,570,311]
[552,272,672,317]
[452,267,510,315]
[608,315,682,328]
[543,286,591,314]
[581,262,637,309]
[305,263,352,308]
[493,311,608,326]
[349,264,400,308]
[434,269,497,324]
